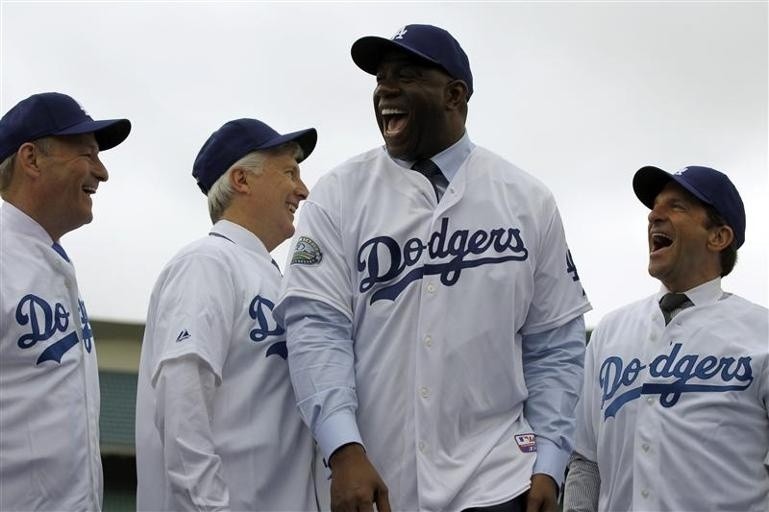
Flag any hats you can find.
[192,118,317,196]
[0,91,131,164]
[633,166,745,252]
[350,23,473,103]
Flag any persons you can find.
[558,162,768,512]
[0,90,133,512]
[132,115,334,512]
[271,21,597,512]
